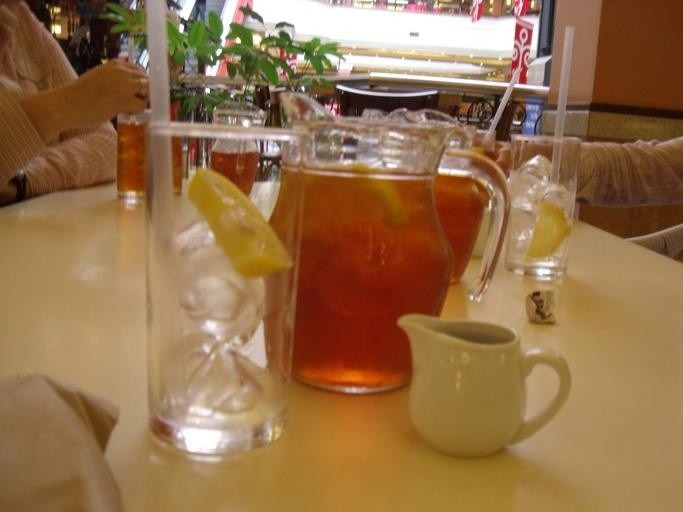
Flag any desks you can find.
[0,179,683,511]
[175,67,552,133]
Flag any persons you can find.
[516,135,683,209]
[0,0,150,207]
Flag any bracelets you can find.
[10,171,27,203]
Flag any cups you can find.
[147,124,306,460]
[117,108,146,207]
[505,134,580,279]
[396,313,570,454]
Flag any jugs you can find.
[262,88,511,395]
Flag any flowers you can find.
[104,6,346,172]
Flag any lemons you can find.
[185,166,296,277]
[524,203,569,261]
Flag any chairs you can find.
[333,82,442,119]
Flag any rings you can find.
[138,79,148,97]
